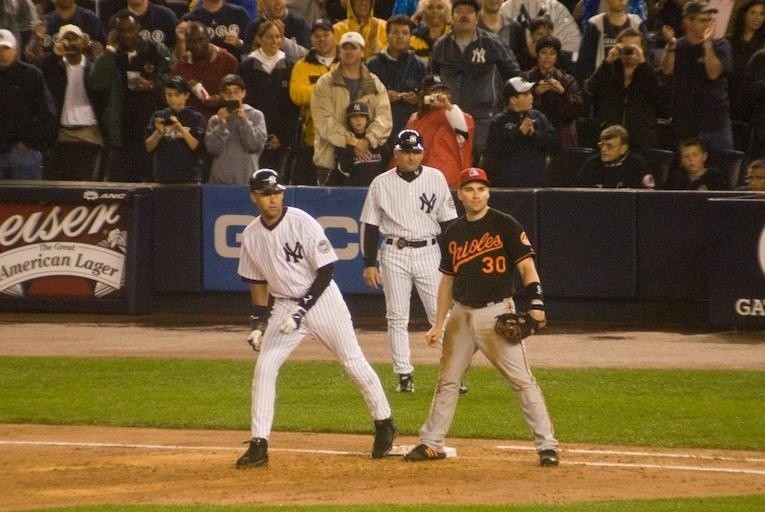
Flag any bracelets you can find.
[524,281,546,302]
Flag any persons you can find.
[235,168,398,468]
[358,129,470,393]
[0,0,763,194]
[402,167,562,466]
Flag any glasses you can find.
[598,142,623,149]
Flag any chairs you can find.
[573,70,746,189]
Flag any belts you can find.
[469,298,504,310]
[386,237,436,249]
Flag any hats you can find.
[167,75,185,90]
[503,76,536,97]
[0,29,16,49]
[311,19,334,33]
[219,74,246,90]
[459,168,489,186]
[339,32,366,48]
[345,103,369,117]
[423,74,451,91]
[59,24,83,41]
[683,0,719,18]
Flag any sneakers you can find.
[372,416,398,458]
[396,374,415,393]
[236,438,269,468]
[403,443,446,461]
[539,450,559,467]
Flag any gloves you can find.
[278,305,308,334]
[247,328,264,352]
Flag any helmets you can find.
[249,169,286,195]
[394,129,424,153]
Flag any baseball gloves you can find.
[495,313,542,347]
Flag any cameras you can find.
[618,47,633,56]
[224,100,241,112]
[423,94,439,106]
[160,118,174,126]
